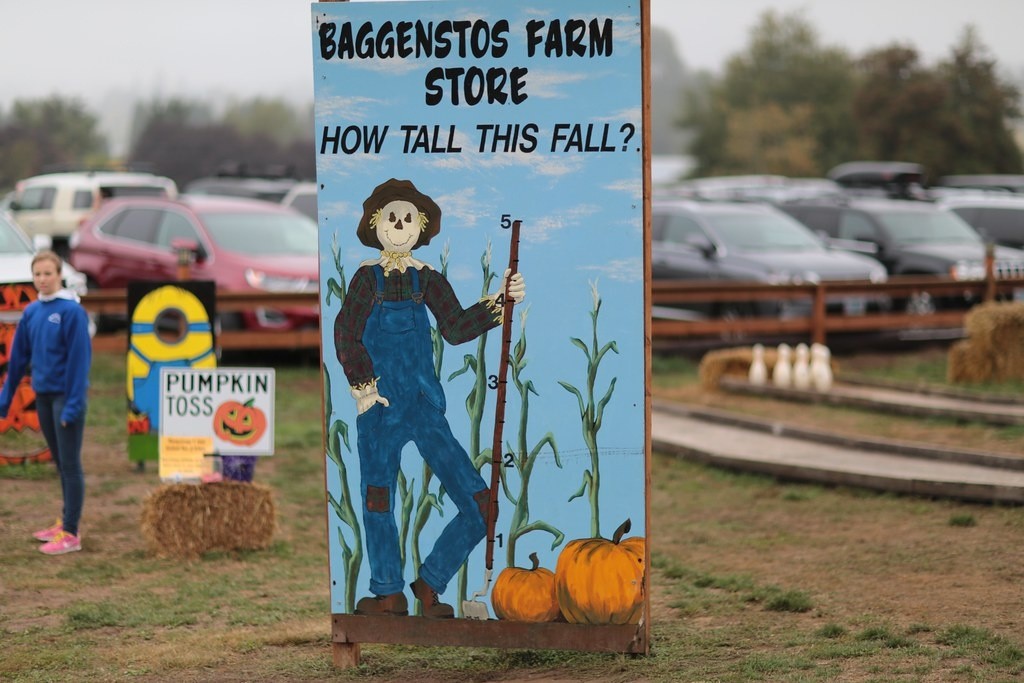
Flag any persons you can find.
[0,247,91,556]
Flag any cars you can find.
[282,185,318,220]
[68,194,321,359]
[667,160,1024,310]
[183,166,299,201]
[0,207,97,380]
[651,199,892,352]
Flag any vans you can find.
[0,172,181,239]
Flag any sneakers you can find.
[33,517,82,555]
